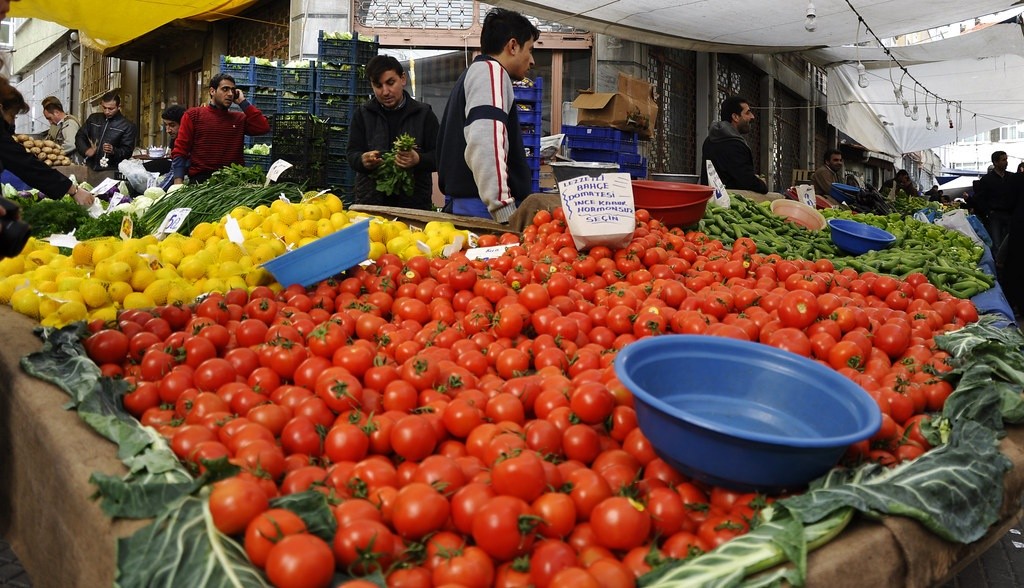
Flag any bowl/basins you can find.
[632,180,718,229]
[615,334,883,498]
[256,215,373,290]
[827,219,896,254]
[651,173,701,184]
[548,161,620,192]
[770,199,827,231]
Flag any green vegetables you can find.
[314,29,377,133]
[274,111,329,184]
[4,191,150,255]
[204,163,267,186]
[224,55,318,108]
[372,132,417,196]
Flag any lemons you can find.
[324,194,343,213]
[0,200,470,331]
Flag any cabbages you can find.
[114,184,185,216]
[244,143,271,155]
[1,181,94,196]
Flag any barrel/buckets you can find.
[832,182,860,205]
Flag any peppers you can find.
[687,192,995,298]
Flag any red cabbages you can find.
[38,192,47,199]
[17,192,34,198]
[97,186,132,203]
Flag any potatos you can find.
[12,135,71,167]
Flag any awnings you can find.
[487,0,1024,54]
[796,10,1024,155]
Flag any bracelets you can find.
[70,185,78,197]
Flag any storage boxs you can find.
[219,32,659,227]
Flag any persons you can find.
[0,73,94,230]
[434,2,541,225]
[811,147,843,195]
[74,90,135,172]
[867,178,872,184]
[139,105,187,174]
[703,97,769,194]
[345,55,438,207]
[879,169,919,197]
[916,185,954,204]
[170,72,271,187]
[950,151,1024,318]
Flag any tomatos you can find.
[82,206,979,588]
